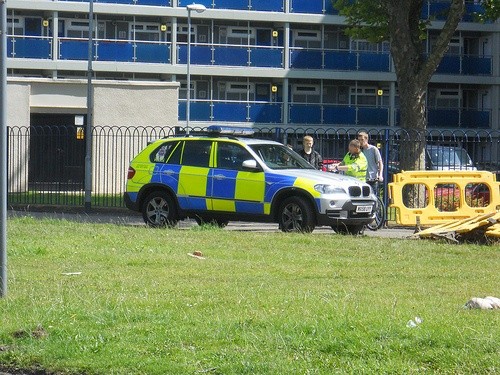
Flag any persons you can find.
[330,139,369,182]
[296,135,323,171]
[358,132,384,196]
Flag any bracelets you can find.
[335,166,338,170]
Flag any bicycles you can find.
[365,178,385,230]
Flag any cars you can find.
[122,126,378,235]
[427,146,480,171]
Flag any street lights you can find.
[187,4,206,136]
